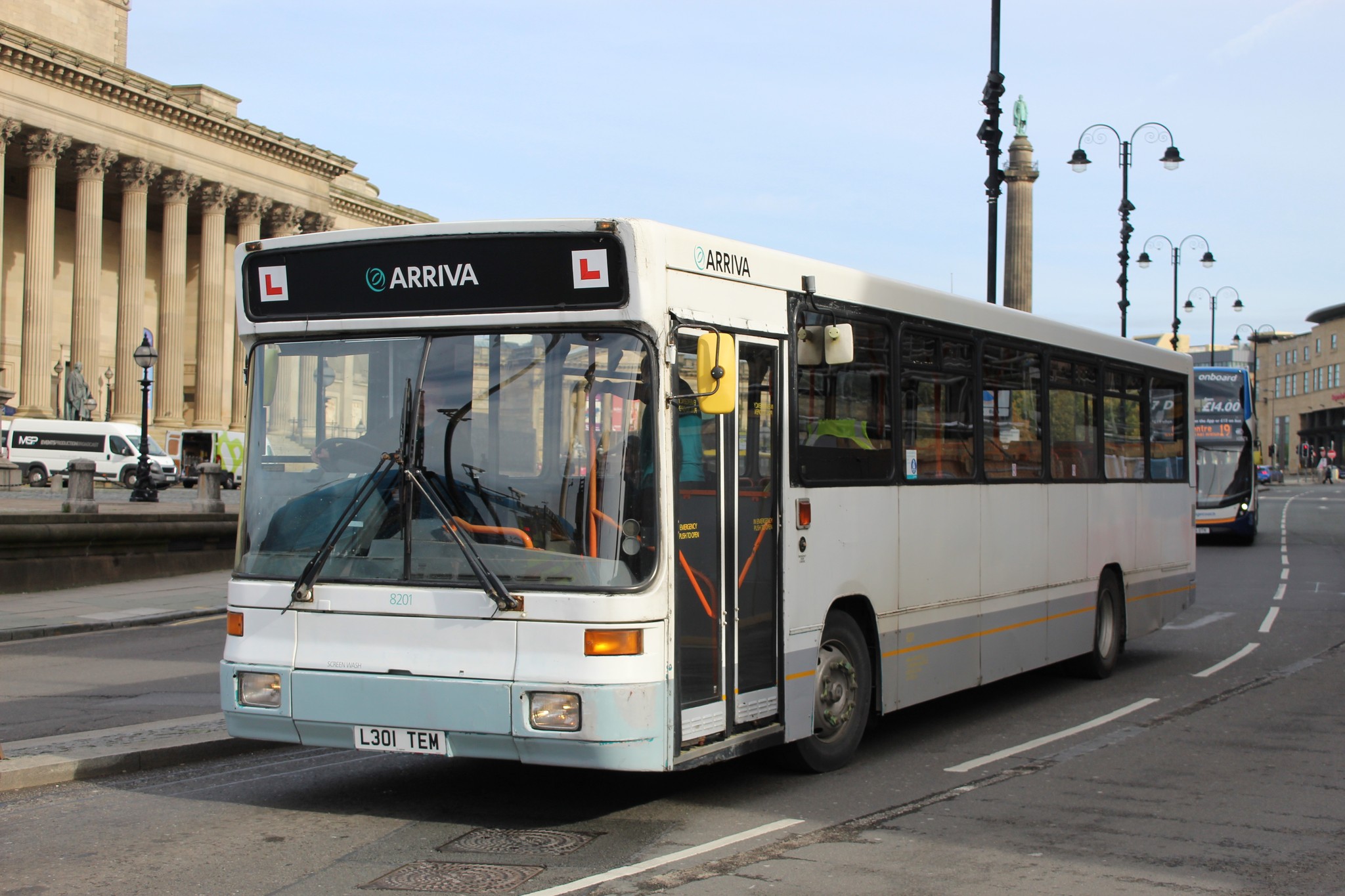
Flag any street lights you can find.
[313,361,335,441]
[1135,234,1216,352]
[1231,324,1279,410]
[133,332,159,502]
[1067,122,1185,337]
[1181,286,1245,366]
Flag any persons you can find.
[584,354,707,487]
[311,373,474,486]
[1322,465,1333,484]
[66,362,91,421]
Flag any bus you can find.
[218,217,1197,776]
[1194,367,1263,546]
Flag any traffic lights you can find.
[1268,445,1274,457]
[1296,443,1313,458]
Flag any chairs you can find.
[811,434,1183,484]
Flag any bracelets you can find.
[603,379,613,393]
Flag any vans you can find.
[6,419,178,490]
[167,430,277,490]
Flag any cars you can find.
[1257,466,1270,484]
